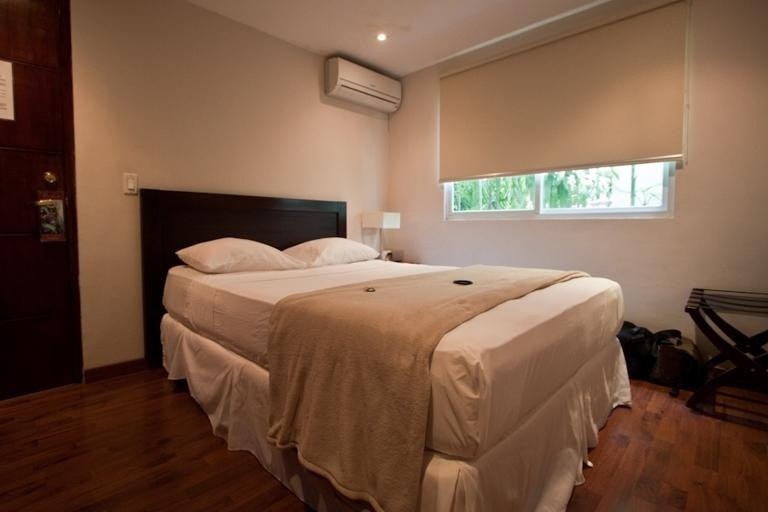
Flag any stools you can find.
[667,285,767,412]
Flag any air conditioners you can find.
[324,55,403,116]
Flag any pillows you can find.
[174,235,379,276]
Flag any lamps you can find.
[361,210,401,260]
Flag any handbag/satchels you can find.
[618,321,702,389]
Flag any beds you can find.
[136,186,626,512]
